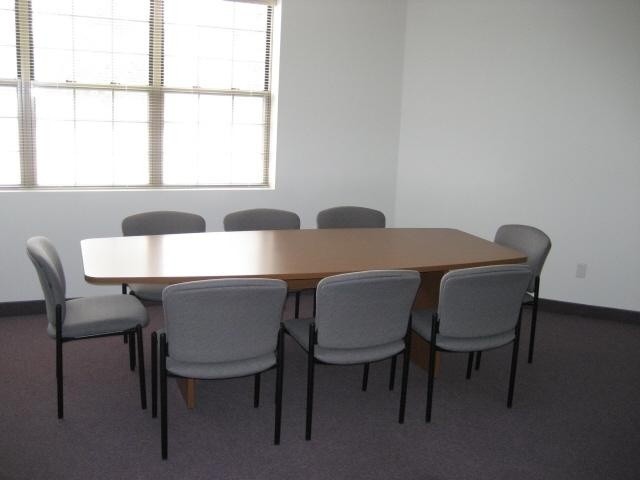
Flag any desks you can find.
[81,228,528,409]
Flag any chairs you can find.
[152,278,288,462]
[26,236,149,419]
[223,208,300,318]
[122,210,205,344]
[281,270,422,440]
[312,206,386,318]
[476,224,552,369]
[391,264,533,424]
